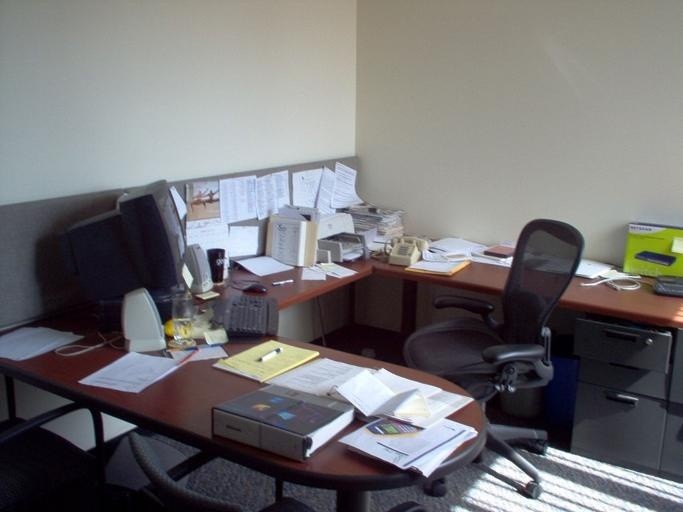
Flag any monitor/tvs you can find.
[57,180,188,325]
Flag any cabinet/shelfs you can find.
[569,313,683,480]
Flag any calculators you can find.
[654,276,683,297]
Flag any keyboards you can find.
[211,295,279,338]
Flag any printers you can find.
[315,212,364,264]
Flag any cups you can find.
[207,248,225,283]
[171,294,193,344]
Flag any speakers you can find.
[121,289,166,354]
[183,244,213,294]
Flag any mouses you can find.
[243,285,267,294]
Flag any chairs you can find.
[402,219,583,498]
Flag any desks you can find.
[373,237,683,417]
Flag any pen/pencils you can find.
[183,343,220,351]
[259,348,282,361]
[273,280,293,286]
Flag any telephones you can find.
[385,237,429,266]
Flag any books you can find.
[211,382,356,463]
[574,259,615,280]
[271,219,308,267]
[265,357,479,478]
[211,339,321,384]
[404,237,516,276]
